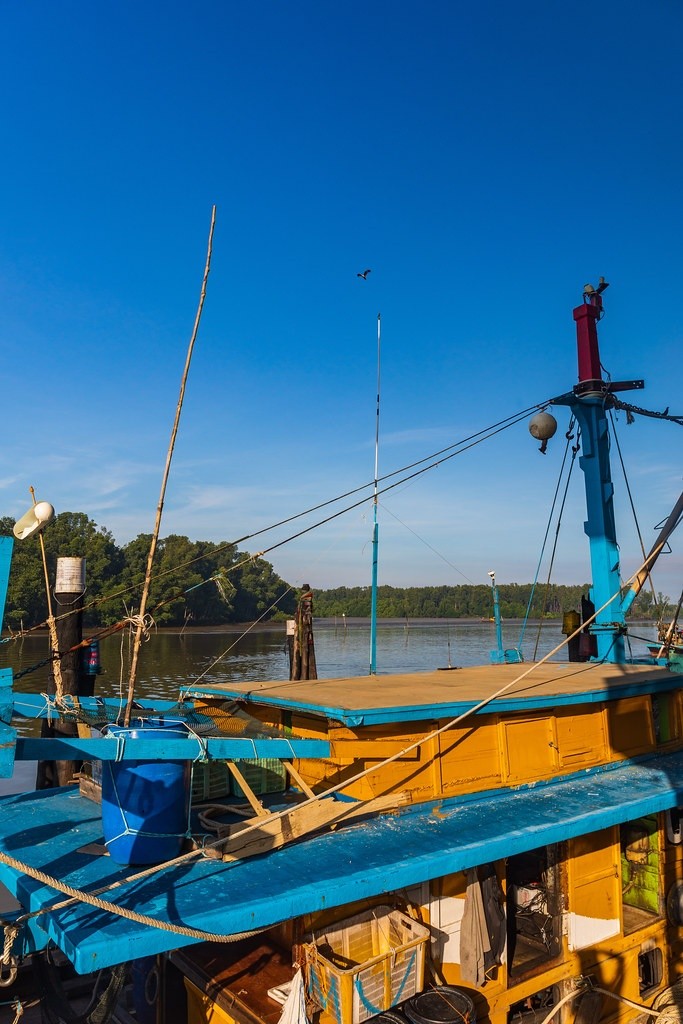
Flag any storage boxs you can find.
[90,726,106,788]
[295,905,431,1024]
[191,758,287,803]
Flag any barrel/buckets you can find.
[101,714,188,866]
[286,620,295,635]
[53,556,87,606]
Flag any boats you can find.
[1,277,683,1024]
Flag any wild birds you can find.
[356,269,372,280]
